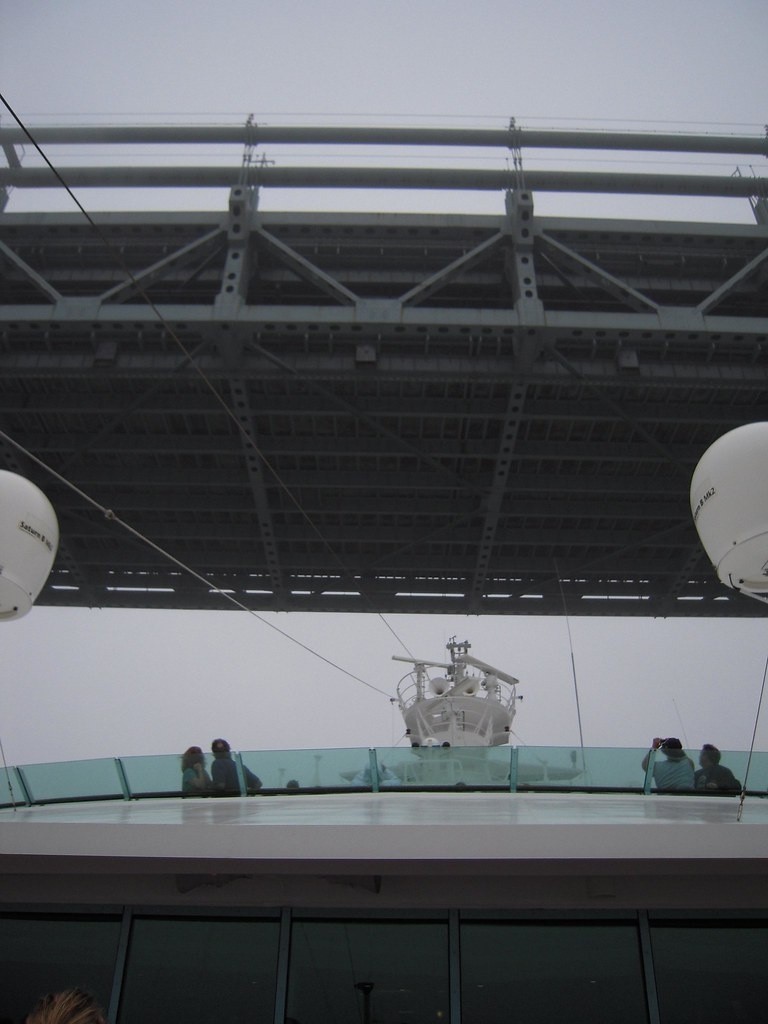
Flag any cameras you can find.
[659,739,666,745]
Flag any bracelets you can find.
[705,783,708,791]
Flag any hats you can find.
[661,737,685,758]
[211,739,230,753]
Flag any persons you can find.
[24,988,111,1024]
[641,737,694,795]
[694,744,742,797]
[206,739,263,797]
[181,747,213,799]
